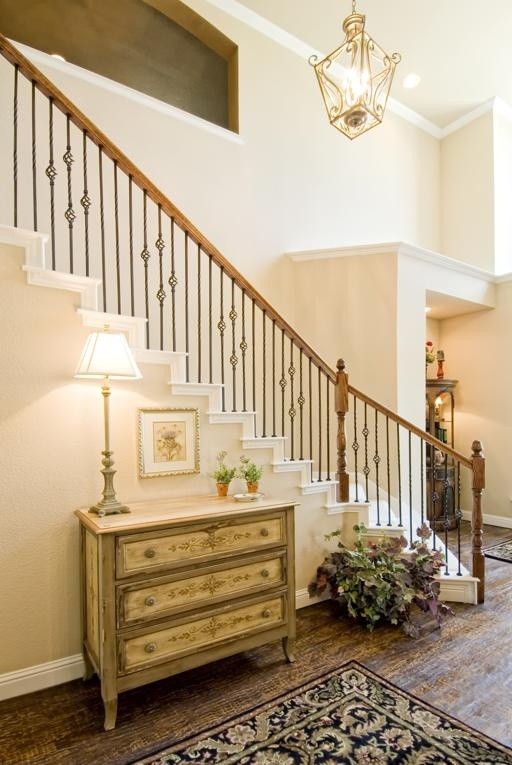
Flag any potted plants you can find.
[206,449,263,498]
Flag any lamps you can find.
[71,321,144,519]
[308,0,403,140]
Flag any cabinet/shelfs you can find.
[424,378,459,532]
[72,492,300,733]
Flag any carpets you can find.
[116,656,511,764]
[483,538,511,563]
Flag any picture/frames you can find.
[135,405,200,479]
